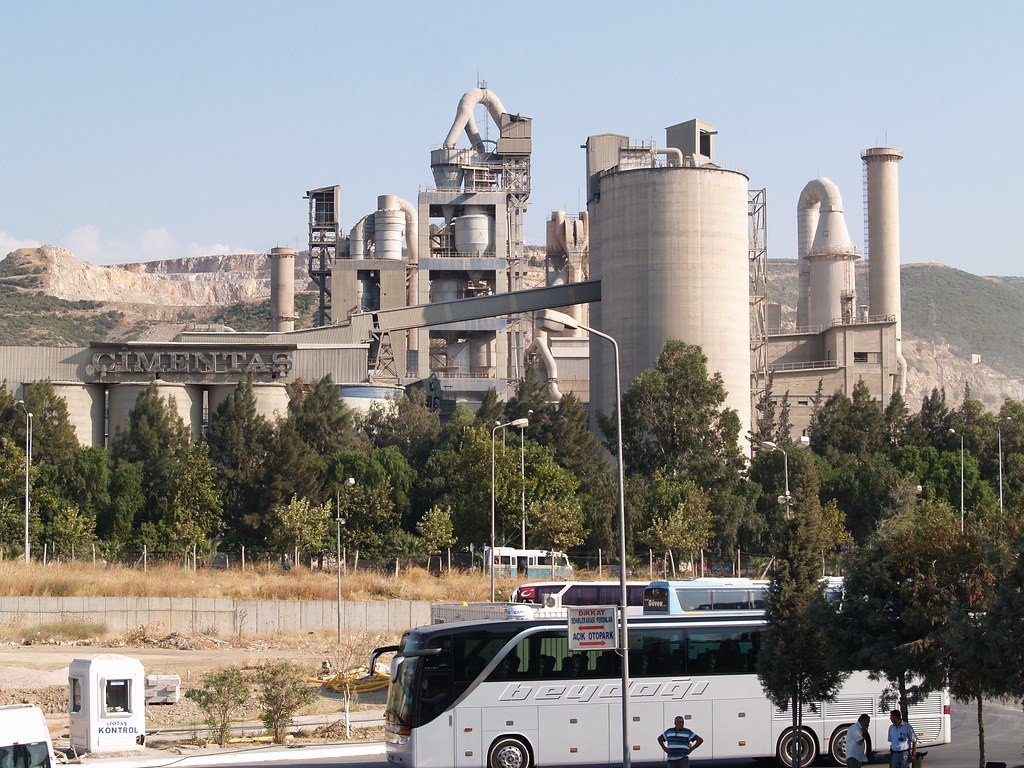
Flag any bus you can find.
[369,613,950,768]
[509,577,651,619]
[642,577,843,642]
[480,547,574,582]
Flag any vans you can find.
[0,705,58,768]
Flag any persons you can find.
[888,710,919,768]
[845,713,870,768]
[658,715,703,768]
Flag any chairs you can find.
[469,646,763,679]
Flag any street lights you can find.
[337,478,355,643]
[533,310,631,768]
[495,420,505,455]
[489,418,530,602]
[18,400,34,562]
[759,440,790,520]
[998,416,1012,512]
[948,429,965,533]
